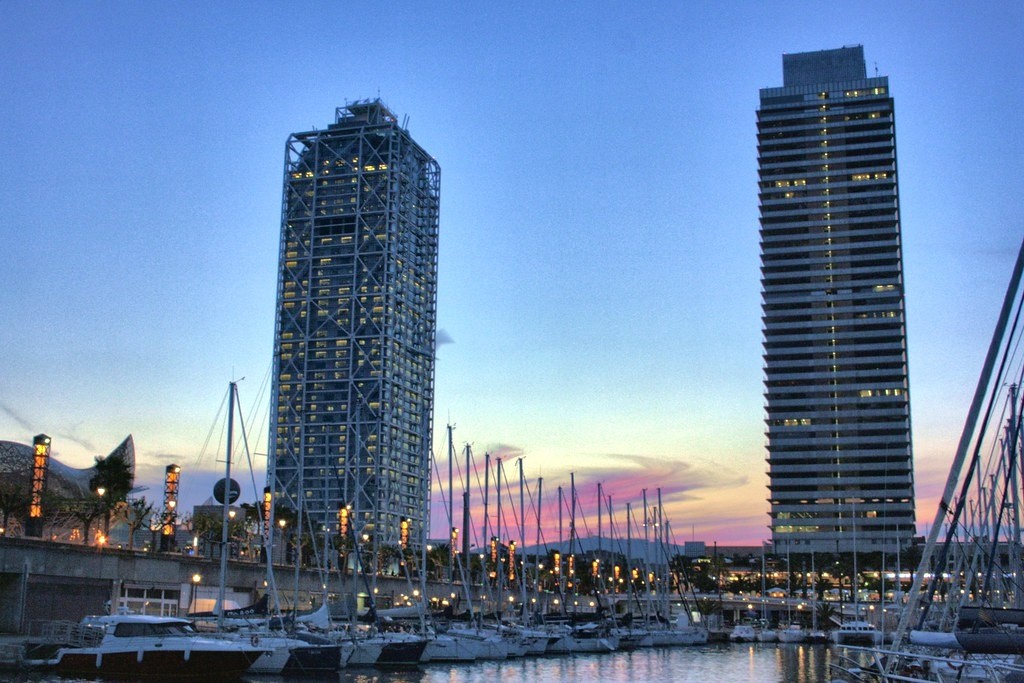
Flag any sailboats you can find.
[17,241,1024,683]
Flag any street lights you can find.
[363,534,369,571]
[228,510,236,559]
[426,544,432,580]
[96,486,106,532]
[168,500,177,553]
[279,519,285,565]
